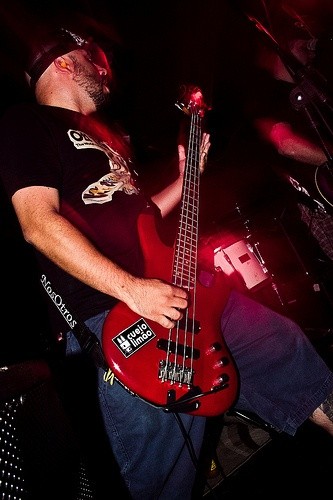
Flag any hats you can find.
[24,23,93,82]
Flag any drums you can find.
[211,241,271,292]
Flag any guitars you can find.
[103,90,240,416]
[314,159,333,207]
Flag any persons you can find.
[240,3,333,291]
[0,25,333,500]
